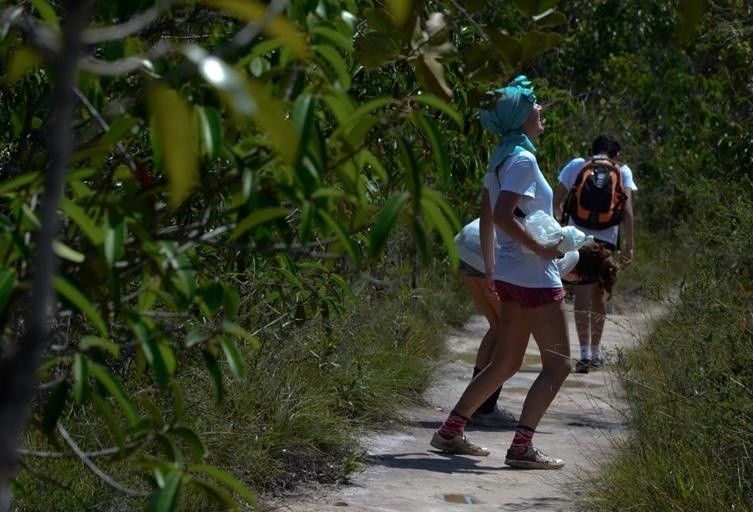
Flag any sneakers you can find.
[430,430,490,457]
[591,354,601,368]
[576,358,591,373]
[505,442,565,470]
[471,404,520,432]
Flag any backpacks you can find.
[562,154,627,231]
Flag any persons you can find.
[430,82,574,469]
[450,216,618,430]
[553,135,637,374]
